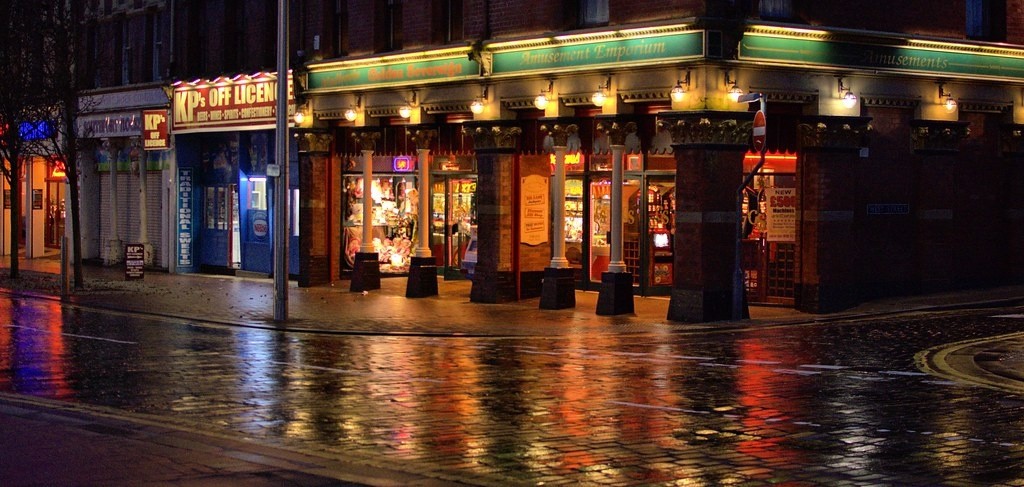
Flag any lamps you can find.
[534,82,553,110]
[470,87,488,114]
[591,77,611,107]
[293,101,310,124]
[838,78,857,108]
[670,70,690,102]
[344,97,361,121]
[399,92,416,119]
[723,76,743,103]
[939,85,956,113]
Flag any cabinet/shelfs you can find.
[341,171,420,280]
